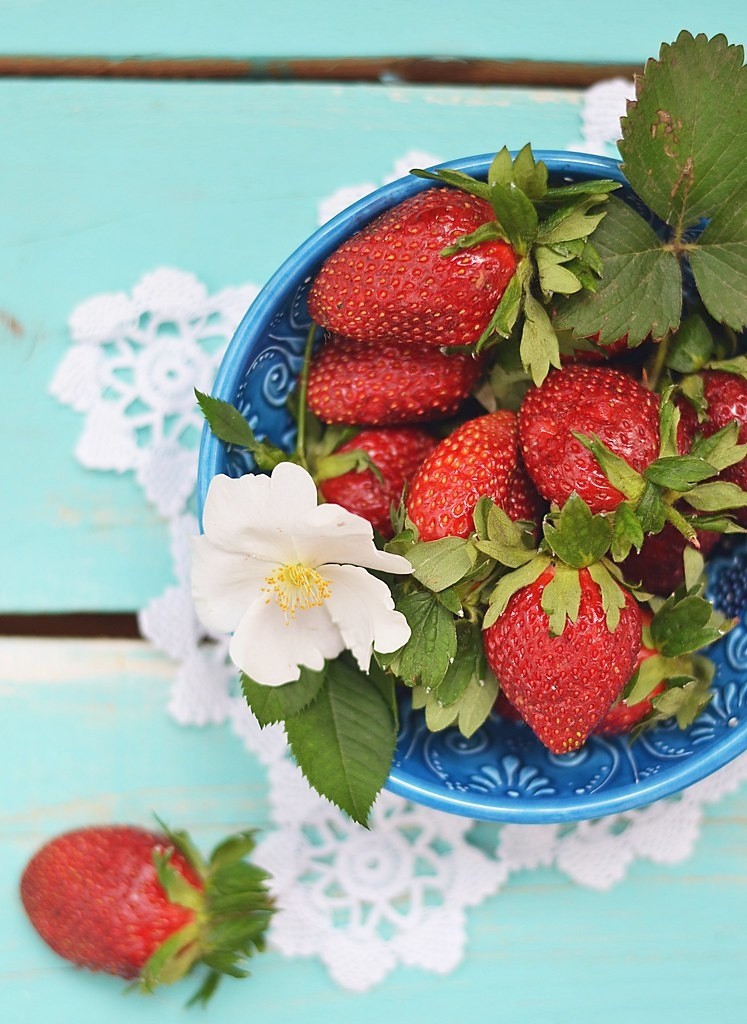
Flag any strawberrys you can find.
[304,189,747,754]
[20,825,288,1010]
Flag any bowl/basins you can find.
[194,149,747,822]
